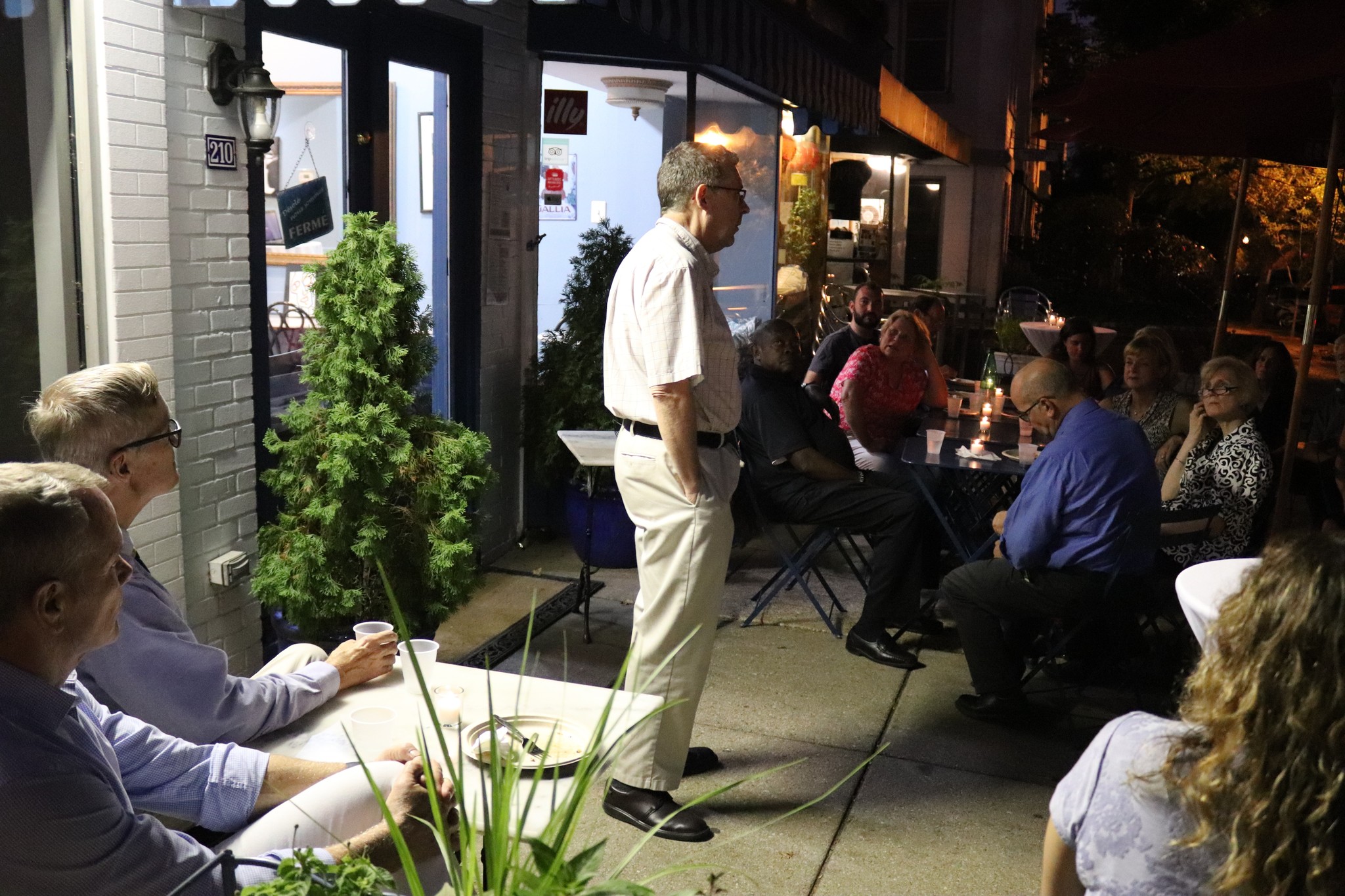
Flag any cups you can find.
[926,429,947,455]
[434,685,464,727]
[990,396,1005,422]
[1049,313,1066,328]
[353,621,395,645]
[996,387,1005,397]
[980,402,993,417]
[947,396,964,418]
[1019,414,1033,436]
[970,432,984,455]
[979,416,991,433]
[397,639,440,693]
[969,392,984,412]
[974,380,981,393]
[1018,443,1038,468]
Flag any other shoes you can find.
[954,690,1028,727]
[1037,652,1124,684]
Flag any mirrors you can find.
[264,83,396,265]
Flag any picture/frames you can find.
[418,112,433,213]
[283,264,319,318]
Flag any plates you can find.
[943,407,980,415]
[460,713,590,770]
[1002,449,1041,462]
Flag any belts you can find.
[623,417,733,449]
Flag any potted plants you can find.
[993,314,1039,374]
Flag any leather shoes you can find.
[845,625,918,668]
[603,782,711,842]
[884,610,945,636]
[682,746,718,776]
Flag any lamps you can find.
[601,76,673,121]
[207,40,285,157]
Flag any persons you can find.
[0,363,467,896]
[735,280,1345,896]
[601,143,744,840]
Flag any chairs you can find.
[812,283,887,356]
[733,429,873,639]
[1019,503,1220,709]
[995,287,1052,321]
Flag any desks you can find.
[841,285,986,379]
[1019,322,1117,357]
[1175,558,1261,648]
[557,430,616,644]
[916,384,1052,448]
[901,437,1027,563]
[258,655,664,896]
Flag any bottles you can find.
[980,346,996,402]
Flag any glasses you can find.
[1018,396,1057,422]
[119,418,183,453]
[1197,386,1241,398]
[692,184,747,206]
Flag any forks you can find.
[493,714,548,756]
